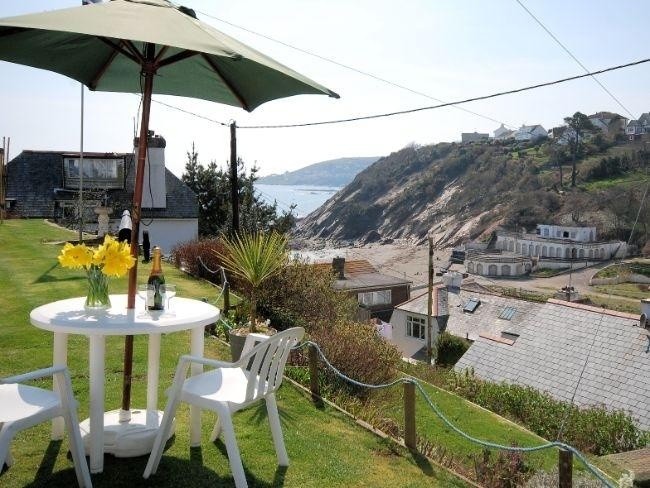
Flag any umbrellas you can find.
[0,0,340,412]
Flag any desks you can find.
[30,294,220,475]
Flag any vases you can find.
[84,270,111,310]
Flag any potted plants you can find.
[212,226,289,369]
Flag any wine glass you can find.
[137,283,156,320]
[158,283,177,318]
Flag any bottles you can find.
[146,245,167,311]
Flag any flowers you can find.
[59,236,137,303]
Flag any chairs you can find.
[142,326,305,488]
[0,365,93,488]
